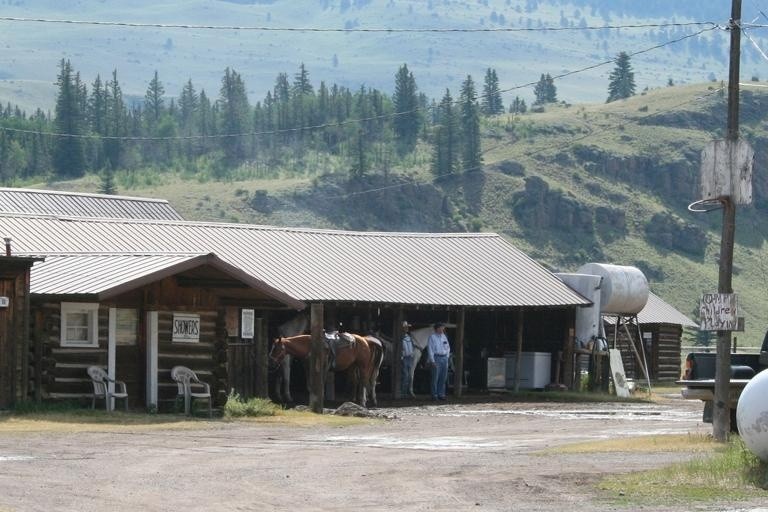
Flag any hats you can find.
[403,321,413,327]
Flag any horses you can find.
[267,332,372,407]
[371,320,448,403]
[266,310,310,405]
[346,334,386,407]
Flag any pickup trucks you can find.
[672,327,766,435]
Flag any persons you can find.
[400,321,416,399]
[427,323,450,399]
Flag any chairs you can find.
[169,365,214,420]
[85,364,130,414]
